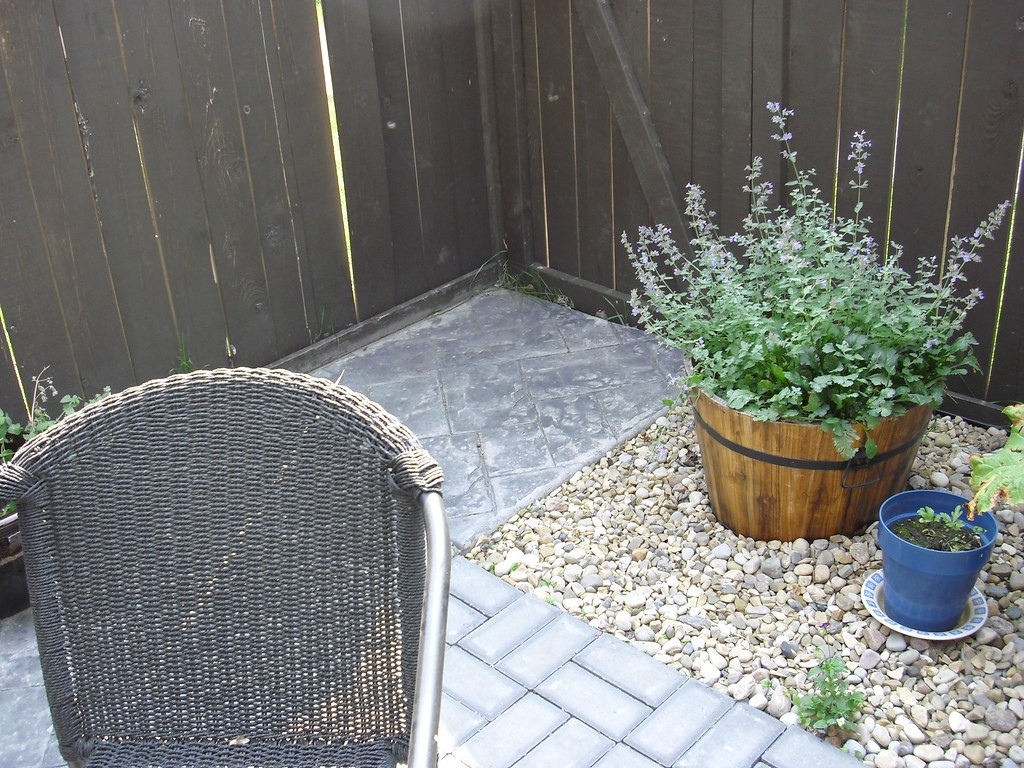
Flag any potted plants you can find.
[877,491,999,632]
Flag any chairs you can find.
[0,365,451,768]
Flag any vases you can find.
[681,358,935,541]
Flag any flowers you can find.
[619,99,1013,462]
